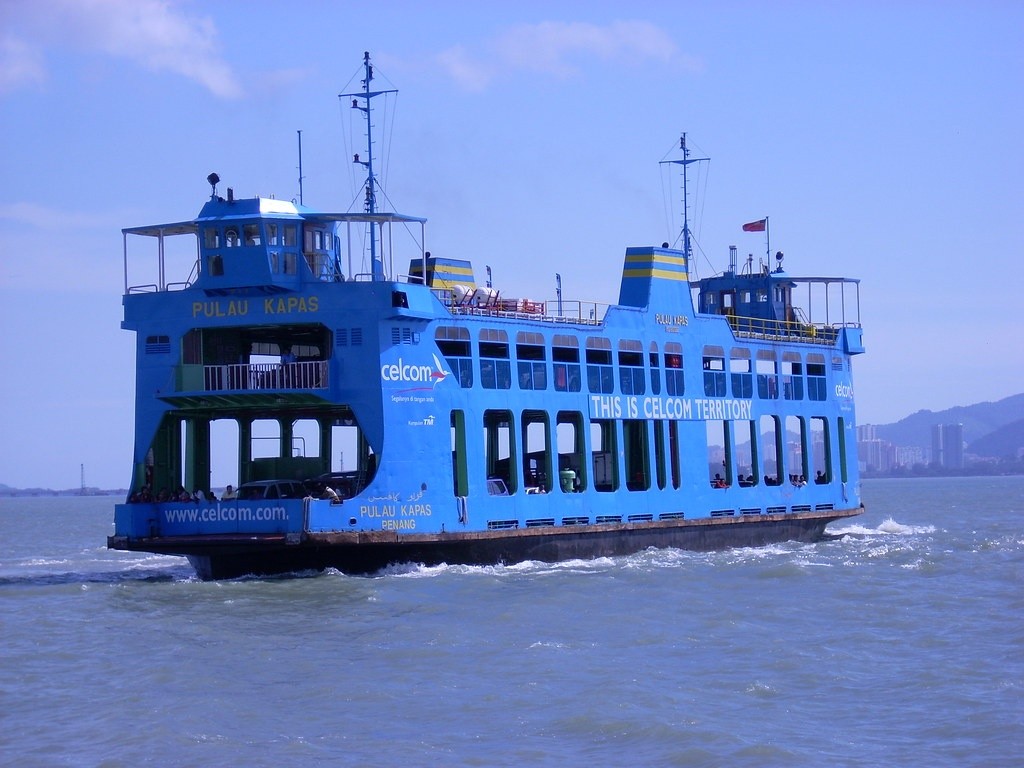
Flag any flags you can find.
[743,219,766,232]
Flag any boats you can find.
[104,42,865,578]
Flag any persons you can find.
[222,486,236,499]
[318,483,340,505]
[250,488,264,498]
[764,475,772,485]
[281,349,296,387]
[789,474,806,486]
[130,486,217,503]
[738,475,752,481]
[245,231,255,246]
[817,471,825,483]
[714,474,729,488]
